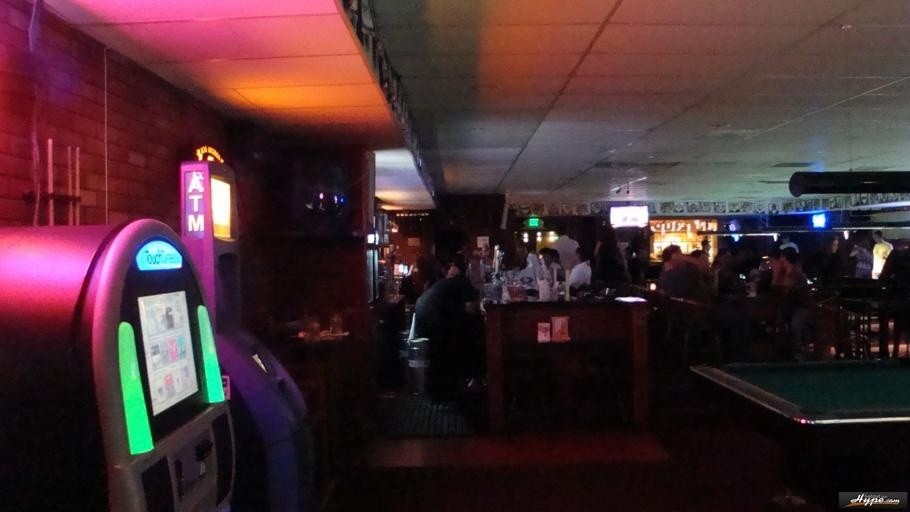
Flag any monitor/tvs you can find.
[129,287,203,423]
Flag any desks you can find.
[485,298,651,433]
[689,358,910,497]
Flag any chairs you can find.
[799,288,844,359]
[635,287,729,369]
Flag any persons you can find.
[412,224,908,401]
